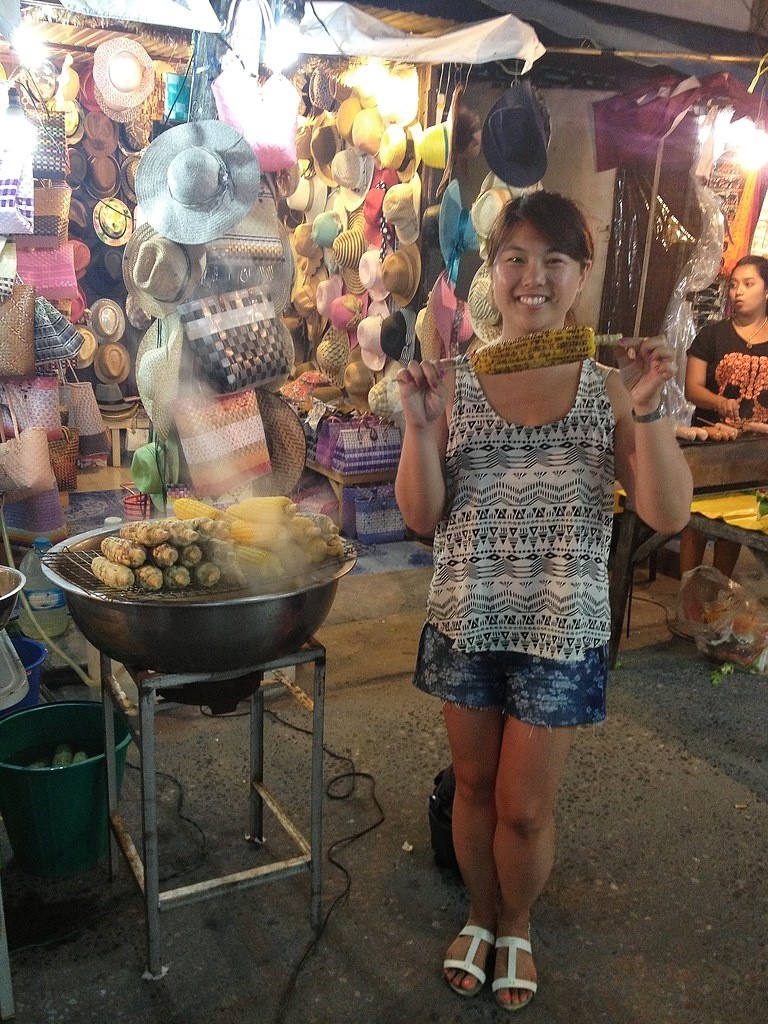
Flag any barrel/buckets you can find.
[0,638,47,717]
[0,700,132,879]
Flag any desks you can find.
[106,418,150,467]
[306,462,397,532]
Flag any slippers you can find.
[492,922,537,1011]
[443,925,494,996]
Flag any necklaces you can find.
[735,317,767,349]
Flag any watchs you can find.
[631,400,667,423]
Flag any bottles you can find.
[19,538,68,638]
[104,517,122,526]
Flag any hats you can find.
[120,225,210,318]
[135,311,193,427]
[134,121,259,247]
[284,58,551,428]
[19,36,153,420]
[130,428,181,511]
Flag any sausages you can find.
[675,422,768,442]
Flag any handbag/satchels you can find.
[300,410,413,540]
[178,284,296,395]
[206,174,285,269]
[211,1,297,172]
[170,389,271,495]
[165,69,192,121]
[0,65,109,546]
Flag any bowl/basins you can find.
[41,518,356,673]
[0,565,26,630]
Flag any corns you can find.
[90,495,346,590]
[469,325,595,375]
[26,742,89,769]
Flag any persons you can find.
[680,256,768,636]
[395,190,694,1007]
